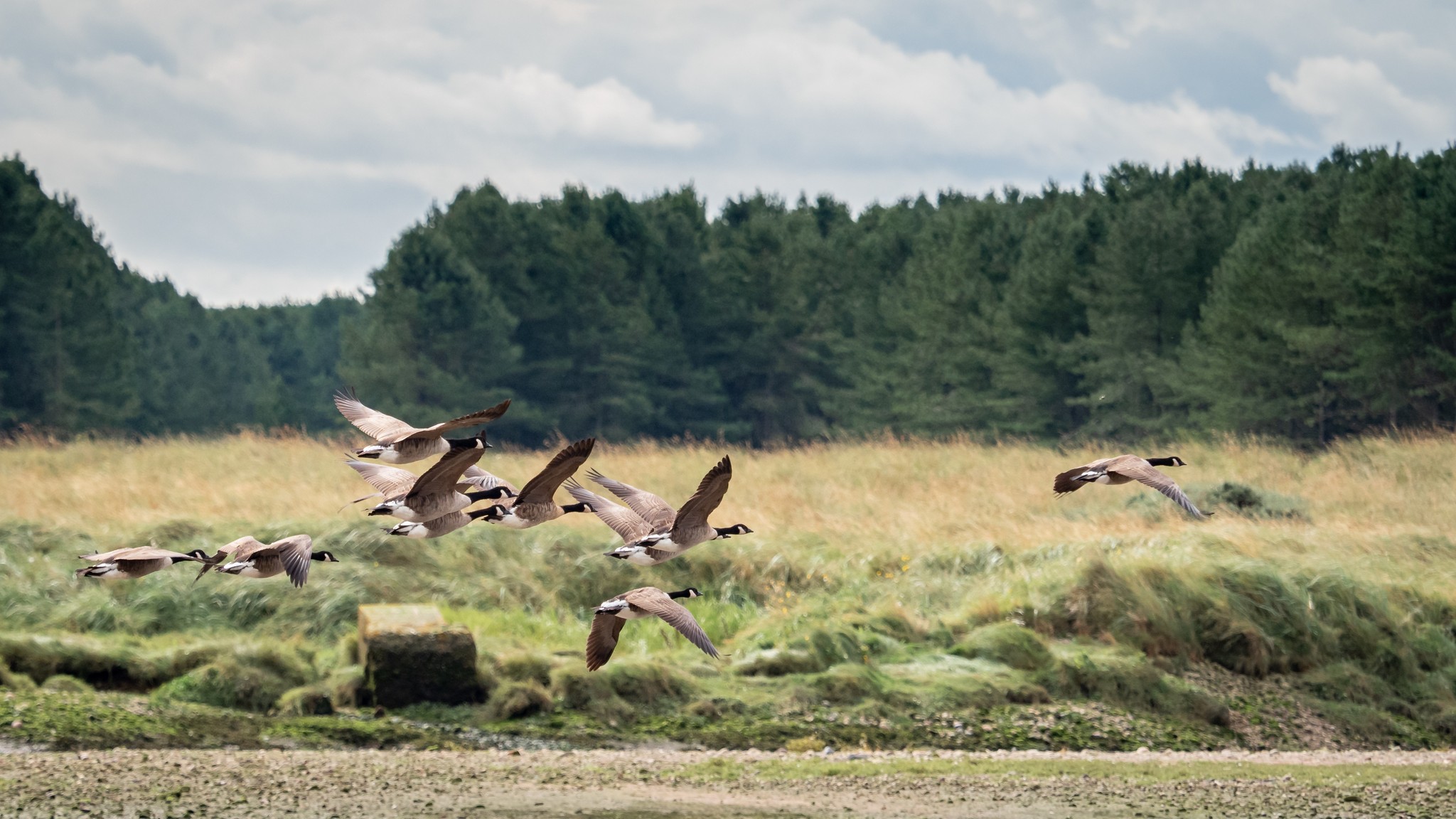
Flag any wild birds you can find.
[192,534,340,588]
[585,454,754,552]
[1052,454,1215,522]
[75,542,209,581]
[561,476,731,566]
[340,450,518,522]
[460,436,598,529]
[337,491,513,539]
[585,587,732,673]
[332,385,511,464]
